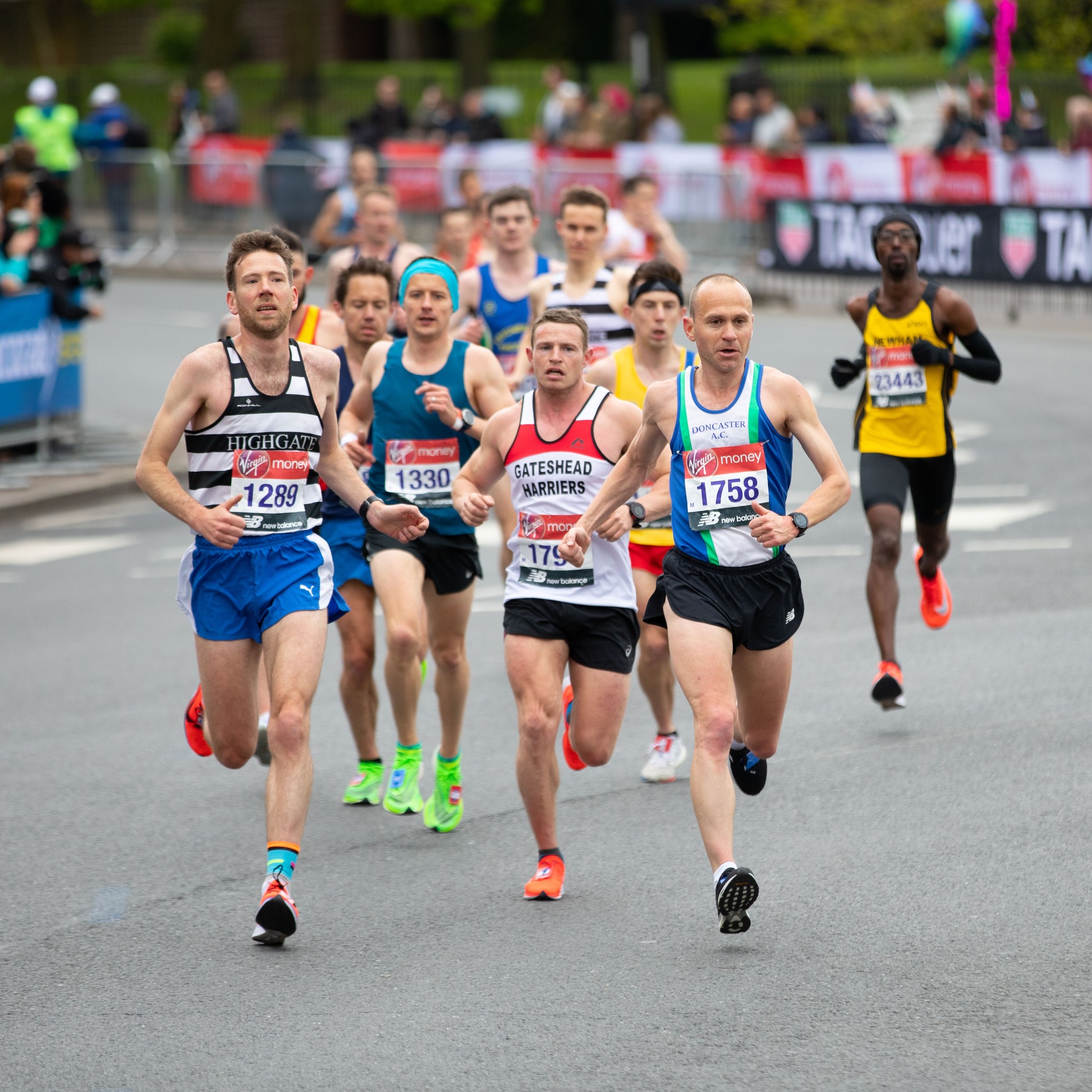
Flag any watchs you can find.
[459,407,476,433]
[625,502,646,528]
[787,512,809,538]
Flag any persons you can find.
[0,69,243,320]
[558,273,852,933]
[449,309,672,899]
[578,260,701,782]
[826,212,1006,712]
[136,228,428,945]
[218,47,1092,586]
[215,230,347,767]
[312,257,426,815]
[338,256,516,836]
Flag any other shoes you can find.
[342,762,384,807]
[424,744,466,833]
[715,867,760,934]
[184,682,222,757]
[523,856,566,900]
[383,744,423,815]
[562,677,591,771]
[252,865,299,948]
[254,712,274,765]
[727,737,769,796]
[871,661,908,712]
[640,735,687,783]
[913,545,952,631]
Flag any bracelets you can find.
[358,496,385,517]
